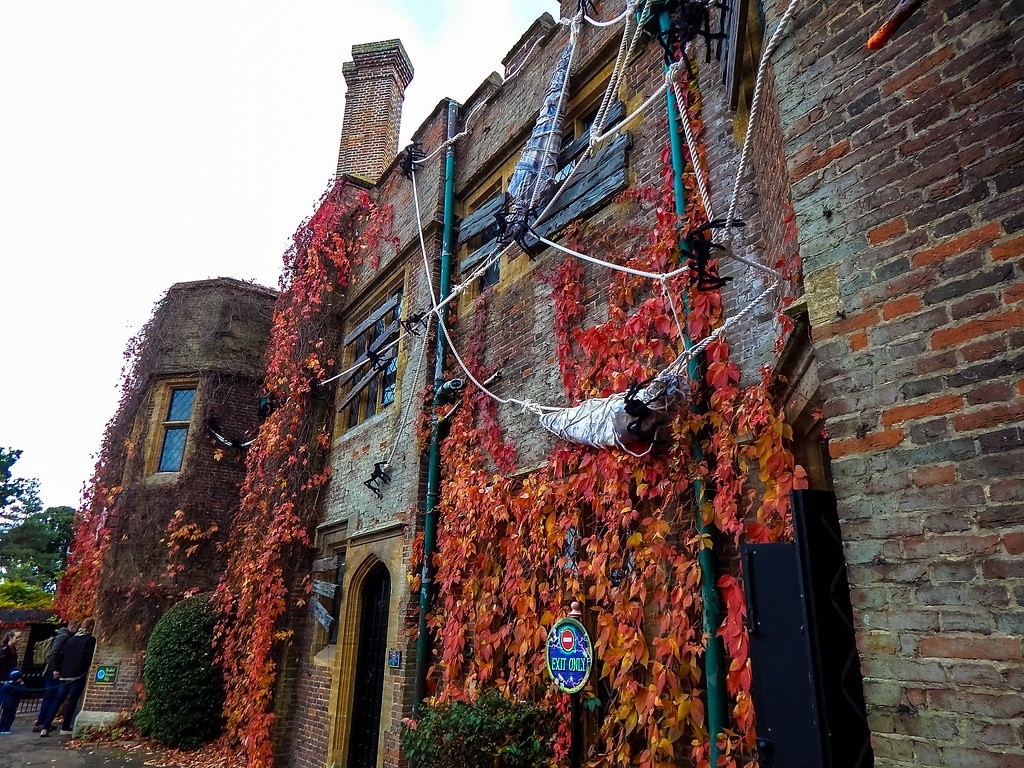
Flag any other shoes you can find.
[60,729,73,734]
[0,730,12,734]
[33,725,43,732]
[48,724,56,731]
[41,729,48,737]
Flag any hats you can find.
[10,671,22,680]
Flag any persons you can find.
[0,671,25,735]
[0,630,21,688]
[40,618,96,737]
[33,618,83,732]
